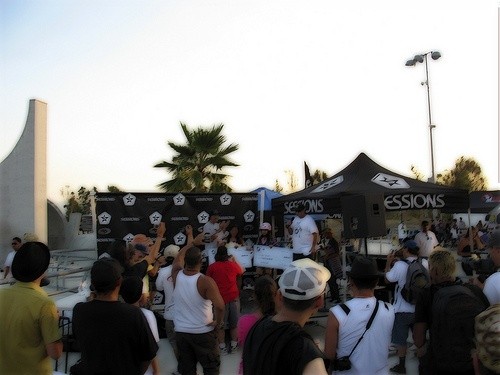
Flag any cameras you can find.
[394,250,403,257]
[204,233,211,244]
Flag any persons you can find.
[323,261,395,375]
[0,242,64,375]
[2,236,22,280]
[385,214,500,375]
[68,257,160,375]
[171,232,225,375]
[239,257,332,375]
[89,202,343,358]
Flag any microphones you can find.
[286,216,295,228]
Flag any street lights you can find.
[403,49,443,185]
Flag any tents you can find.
[270,152,473,260]
[247,187,286,214]
[469,189,500,214]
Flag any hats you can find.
[400,240,419,249]
[294,204,306,212]
[259,222,272,231]
[165,245,180,259]
[278,258,331,299]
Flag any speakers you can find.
[340,188,387,240]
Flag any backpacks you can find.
[400,257,431,305]
